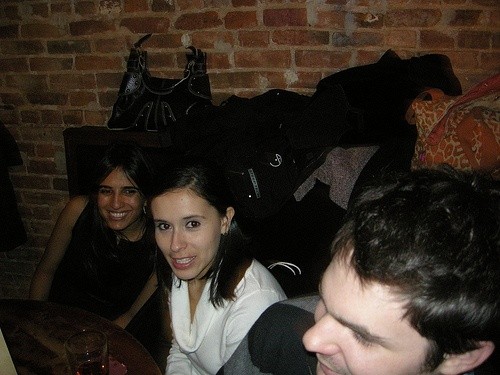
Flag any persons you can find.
[215,162,500,375]
[29,145,163,359]
[146,158,289,375]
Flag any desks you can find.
[0,296,163,375]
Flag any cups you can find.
[65,331,109,375]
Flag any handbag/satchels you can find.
[405,72,500,174]
[106,34,213,133]
